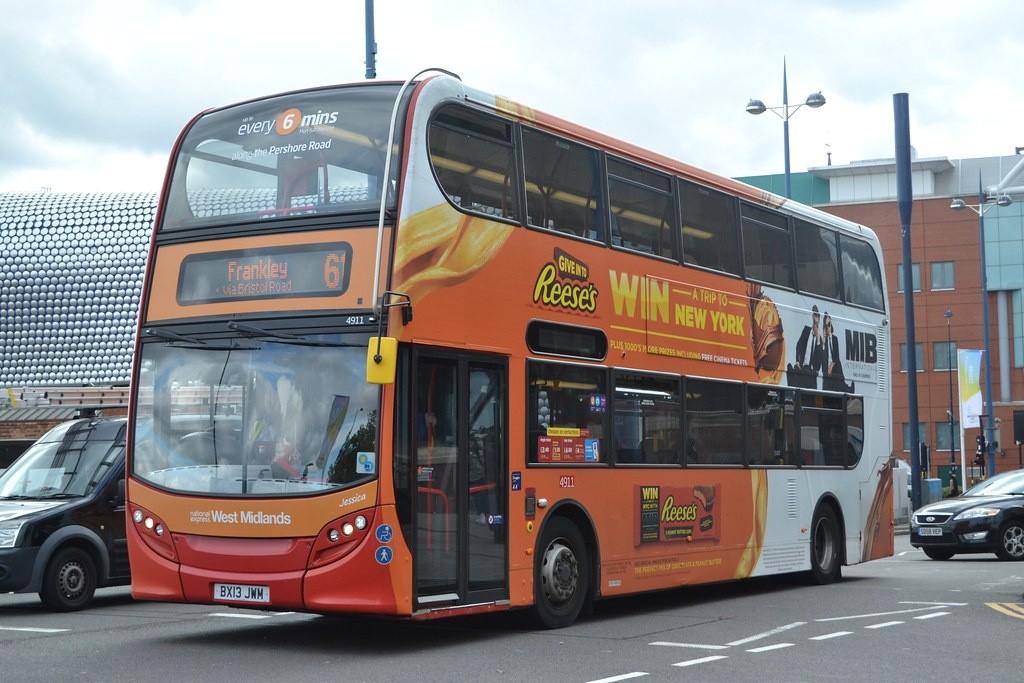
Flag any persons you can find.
[949,474,956,497]
[786,306,854,394]
[247,378,442,465]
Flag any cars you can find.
[0,411,244,613]
[908,468,1024,561]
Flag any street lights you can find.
[949,167,1013,479]
[943,308,960,498]
[744,54,827,199]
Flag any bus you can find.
[124,66,897,632]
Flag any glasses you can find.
[813,319,819,328]
[824,323,831,328]
